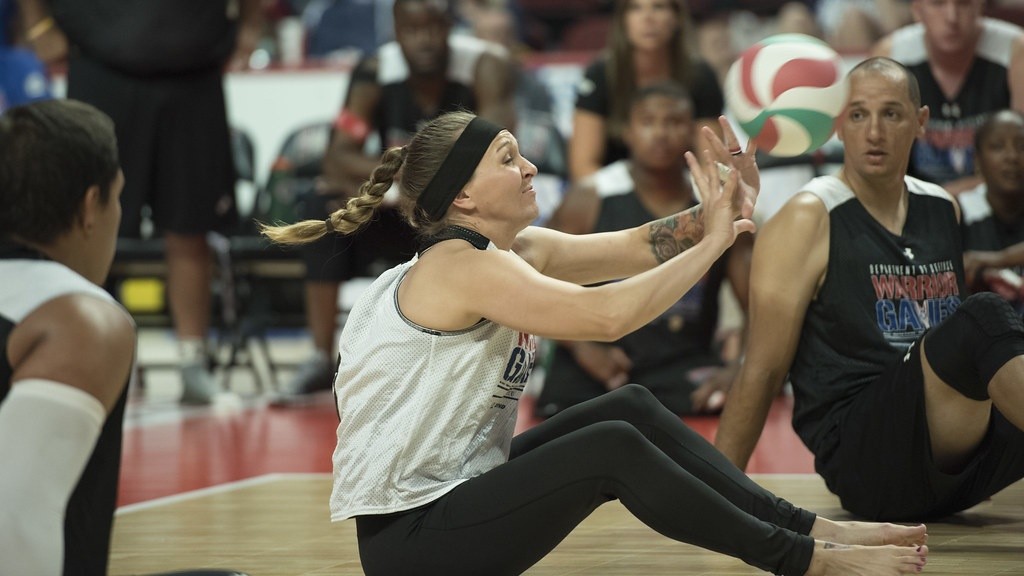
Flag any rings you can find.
[729,148,742,154]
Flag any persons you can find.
[0,99,140,576]
[15,0,282,408]
[714,56,1024,522]
[288,0,913,399]
[956,109,1024,307]
[873,0,1024,199]
[259,112,929,576]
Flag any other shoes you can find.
[180,367,212,405]
[266,358,336,406]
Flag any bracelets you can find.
[24,17,55,42]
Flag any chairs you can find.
[126,126,336,403]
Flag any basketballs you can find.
[725,28,850,158]
